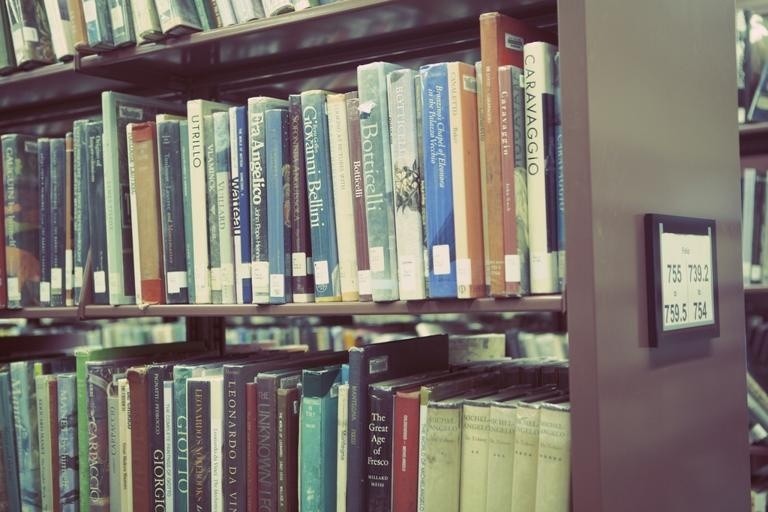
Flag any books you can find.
[748,60,768,121]
[743,168,768,287]
[747,369,768,445]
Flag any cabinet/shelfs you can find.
[2,0,752,512]
